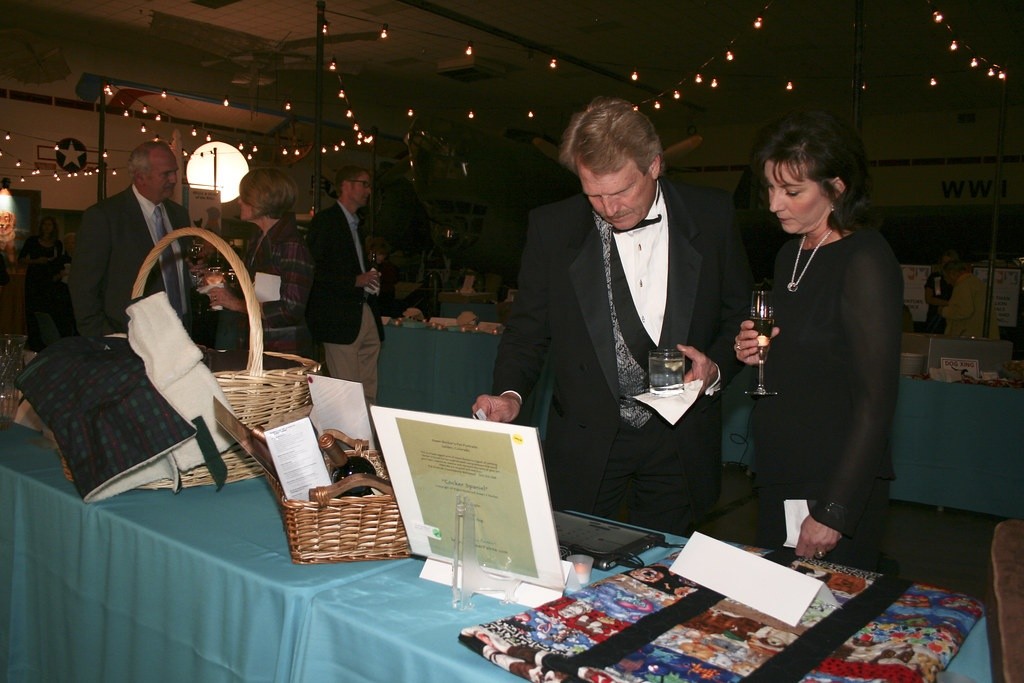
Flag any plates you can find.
[900,351,924,376]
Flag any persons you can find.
[0,215,400,368]
[308,164,388,405]
[208,167,317,359]
[471,97,753,539]
[67,141,228,340]
[733,112,905,565]
[901,250,999,339]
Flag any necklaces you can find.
[787,227,833,292]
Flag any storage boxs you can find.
[926,334,1014,374]
[402,321,424,329]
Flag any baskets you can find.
[261,429,411,564]
[57,228,320,499]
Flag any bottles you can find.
[319,433,383,496]
[366,252,379,274]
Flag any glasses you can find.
[346,179,372,189]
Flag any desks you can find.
[0,301,1024,683]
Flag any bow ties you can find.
[612,188,661,234]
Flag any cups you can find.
[566,554,593,584]
[648,349,685,396]
[205,267,223,286]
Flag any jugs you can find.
[0,333,29,431]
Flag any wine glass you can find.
[190,243,204,277]
[744,289,780,395]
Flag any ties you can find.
[154,206,182,323]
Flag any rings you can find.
[735,342,742,351]
[817,550,825,557]
[214,297,217,301]
